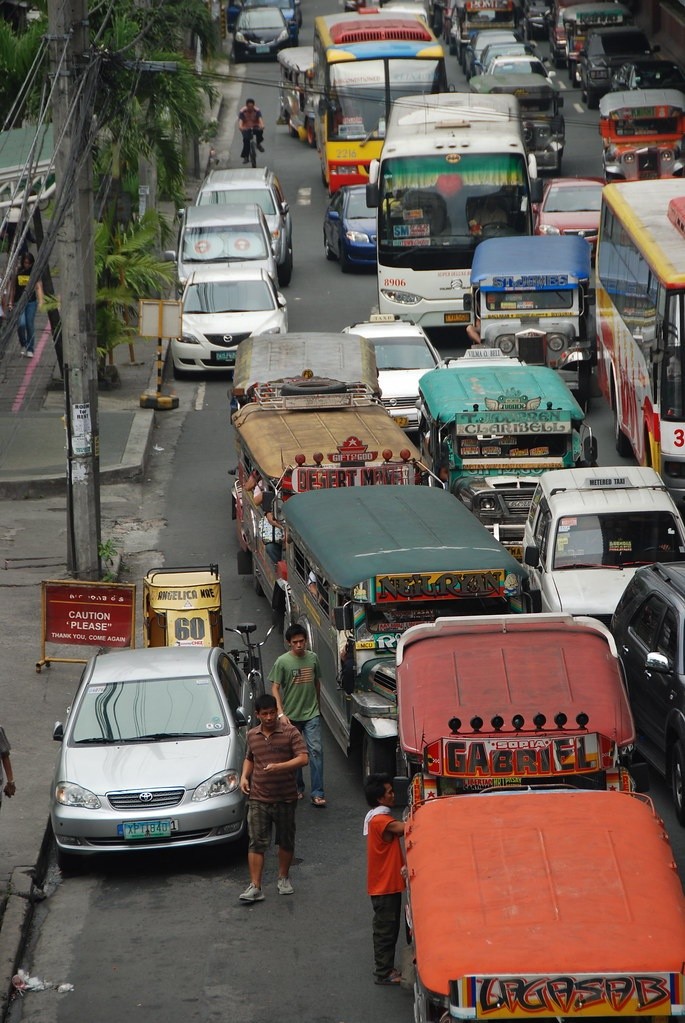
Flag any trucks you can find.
[274,45,317,146]
[468,72,685,182]
[462,234,594,410]
[228,329,685,1023]
[415,365,598,563]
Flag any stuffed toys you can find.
[469,219,482,235]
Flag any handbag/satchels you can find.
[259,516,285,544]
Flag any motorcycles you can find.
[143,564,224,649]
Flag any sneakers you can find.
[239,883,265,901]
[277,876,295,894]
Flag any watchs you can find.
[7,781,15,786]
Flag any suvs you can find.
[553,0,662,109]
[163,166,292,291]
[609,560,685,826]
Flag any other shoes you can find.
[257,144,265,152]
[20,346,26,355]
[374,969,402,985]
[26,351,33,358]
[243,156,249,164]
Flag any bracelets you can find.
[276,713,283,720]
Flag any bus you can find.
[313,7,447,197]
[593,178,685,514]
[366,94,535,331]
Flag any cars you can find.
[226,0,556,81]
[532,178,605,257]
[172,268,442,435]
[609,60,685,95]
[50,645,254,854]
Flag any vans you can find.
[521,466,685,629]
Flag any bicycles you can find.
[240,126,261,168]
[225,624,277,699]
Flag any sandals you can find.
[311,797,327,807]
[298,792,304,800]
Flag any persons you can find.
[339,629,356,702]
[4,190,30,261]
[364,773,406,986]
[244,468,269,506]
[8,251,43,358]
[238,694,310,901]
[471,194,508,229]
[0,727,16,809]
[268,624,326,807]
[307,570,324,605]
[264,508,283,564]
[239,98,265,164]
[0,277,7,363]
